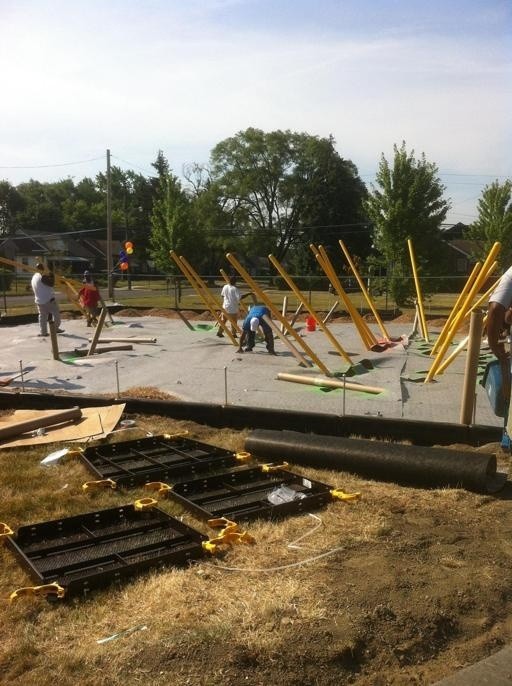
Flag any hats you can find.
[250,318,259,332]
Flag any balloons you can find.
[120,256,128,263]
[125,241,134,249]
[126,247,133,255]
[121,262,130,270]
[119,250,126,258]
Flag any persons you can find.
[77,273,102,327]
[217,277,242,339]
[83,269,90,282]
[235,306,278,357]
[485,265,511,445]
[30,262,66,337]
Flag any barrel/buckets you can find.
[306,316,316,331]
